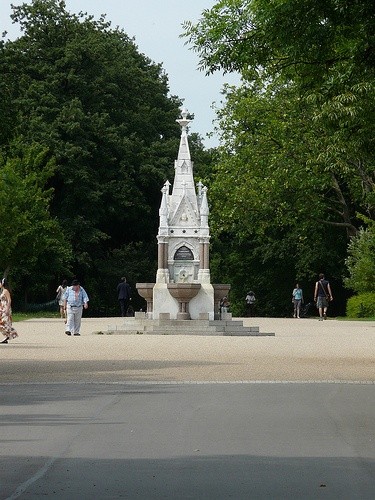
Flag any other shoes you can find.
[293,314,296,317]
[324,313,327,320]
[0,340,8,343]
[297,316,300,318]
[74,333,80,336]
[320,318,323,321]
[65,331,72,335]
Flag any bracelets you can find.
[314,297,316,298]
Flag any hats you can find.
[71,280,81,285]
[247,291,255,294]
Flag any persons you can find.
[293,283,304,319]
[245,290,256,317]
[314,274,334,321]
[56,279,68,319]
[0,277,17,344]
[116,276,132,317]
[63,279,89,336]
[220,297,230,313]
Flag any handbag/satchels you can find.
[326,295,330,302]
